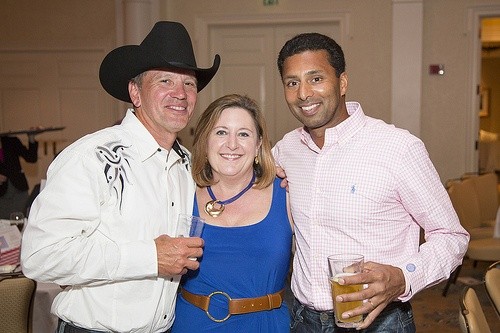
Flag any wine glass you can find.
[10,212,25,232]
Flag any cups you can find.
[327,253,363,328]
[175,214,204,260]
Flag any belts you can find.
[178,288,286,322]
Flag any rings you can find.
[368,300,375,310]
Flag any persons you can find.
[269,32,471,333]
[168,93,294,332]
[19,20,289,333]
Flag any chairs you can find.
[457,284,492,333]
[460,172,500,268]
[484,263,500,318]
[0,272,38,333]
[442,179,500,297]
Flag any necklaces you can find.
[204,169,256,219]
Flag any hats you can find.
[99,21,221,103]
